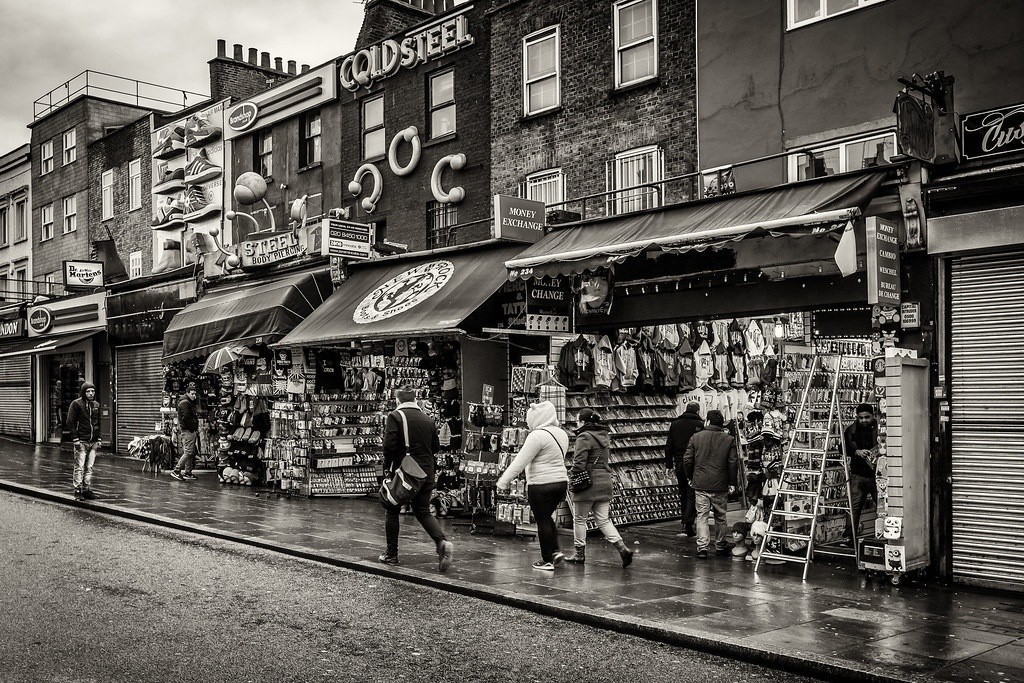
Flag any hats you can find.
[424,376,465,518]
[127,436,151,460]
[222,467,254,482]
[217,365,232,467]
[730,358,783,543]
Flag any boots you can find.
[613,540,633,568]
[564,546,585,565]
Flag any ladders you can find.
[753,354,860,580]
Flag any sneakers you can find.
[532,559,554,570]
[148,115,223,275]
[170,471,184,481]
[183,473,198,479]
[552,552,564,565]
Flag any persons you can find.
[682,410,738,559]
[663,400,706,538]
[493,400,569,572]
[376,384,454,574]
[564,409,635,570]
[65,381,102,501]
[573,268,615,315]
[838,402,878,548]
[747,410,763,427]
[169,387,199,482]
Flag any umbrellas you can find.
[232,343,261,358]
[199,343,241,376]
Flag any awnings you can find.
[267,245,531,348]
[0,328,103,357]
[504,166,890,282]
[160,268,331,366]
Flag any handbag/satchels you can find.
[569,470,592,493]
[379,455,428,511]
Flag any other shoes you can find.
[75,491,84,501]
[694,546,708,557]
[682,524,695,537]
[840,538,853,548]
[437,540,453,572]
[83,491,99,499]
[378,552,399,566]
[716,548,730,555]
